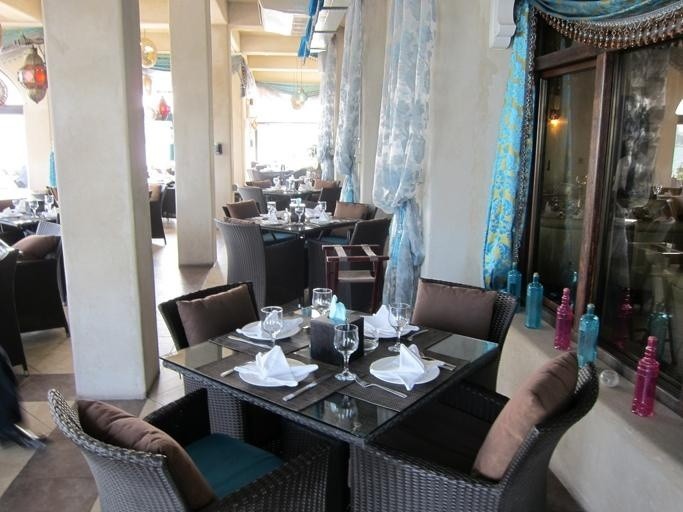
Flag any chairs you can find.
[156,280,261,444]
[213,216,305,308]
[46,385,330,510]
[409,276,520,392]
[311,199,377,245]
[346,360,598,512]
[233,162,342,200]
[0,185,70,377]
[222,199,262,220]
[147,168,175,245]
[262,200,333,211]
[306,217,392,305]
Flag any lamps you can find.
[290,58,307,110]
[549,84,561,125]
[13,32,47,104]
[157,97,170,120]
[139,28,158,96]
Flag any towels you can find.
[358,303,420,338]
[232,344,319,388]
[393,342,446,393]
[232,316,303,342]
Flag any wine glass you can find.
[273,177,279,187]
[28,199,38,222]
[311,288,332,318]
[333,324,359,382]
[295,204,304,226]
[267,201,276,215]
[260,305,283,348]
[44,195,54,212]
[317,200,327,219]
[386,302,410,353]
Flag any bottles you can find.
[506,261,520,314]
[599,369,619,388]
[645,302,667,362]
[630,336,659,418]
[523,273,543,329]
[575,303,600,371]
[553,288,573,354]
[612,286,632,352]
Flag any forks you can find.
[353,375,408,399]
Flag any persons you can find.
[0,347,52,452]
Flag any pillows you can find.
[334,201,370,220]
[73,397,218,511]
[223,217,254,224]
[410,277,497,341]
[468,351,578,481]
[174,284,258,348]
[225,200,259,219]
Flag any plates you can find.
[362,330,410,339]
[238,357,310,388]
[241,320,301,341]
[368,355,441,386]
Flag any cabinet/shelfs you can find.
[624,235,682,370]
[321,243,389,313]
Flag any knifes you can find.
[281,371,335,402]
